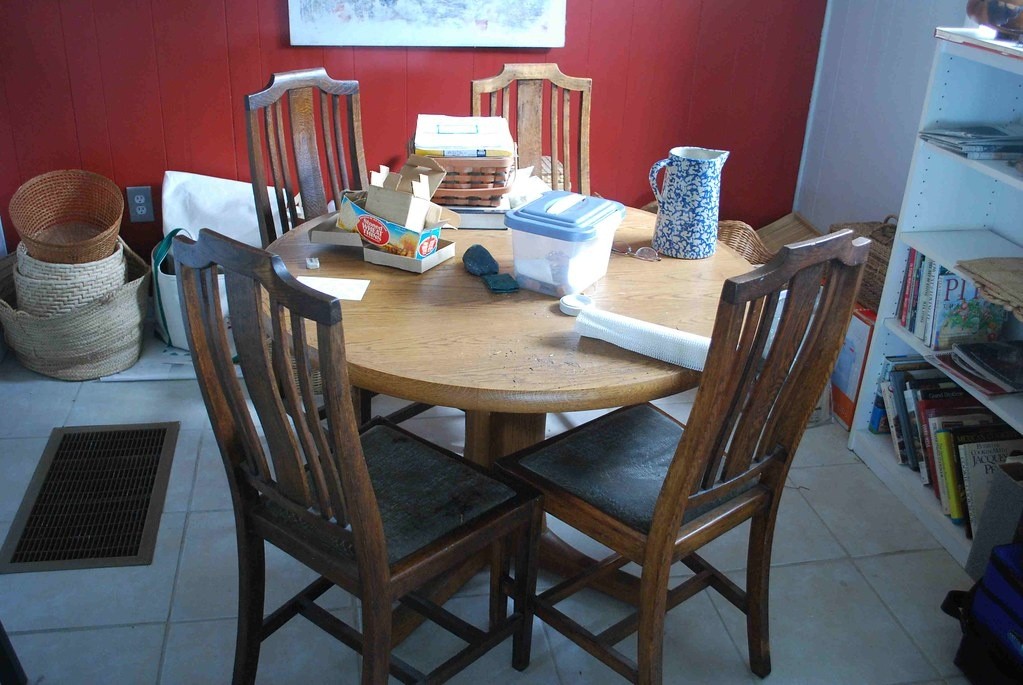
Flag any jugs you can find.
[648,146,731,260]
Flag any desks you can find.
[255,206,759,573]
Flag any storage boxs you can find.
[337,189,462,259]
[828,220,897,313]
[307,211,456,274]
[831,301,878,431]
[364,153,446,232]
[505,191,626,296]
[952,544,1023,685]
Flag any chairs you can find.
[471,62,591,196]
[173,229,544,685]
[243,65,436,455]
[489,229,872,685]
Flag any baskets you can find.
[0,242,154,383]
[824,215,898,315]
[407,127,516,206]
[718,220,778,264]
[10,170,125,264]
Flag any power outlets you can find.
[126,186,155,223]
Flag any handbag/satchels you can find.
[152,228,238,358]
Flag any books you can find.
[934,26,1023,59]
[897,248,1006,349]
[869,353,1023,542]
[925,340,1023,396]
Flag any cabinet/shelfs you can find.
[848,26,1022,580]
